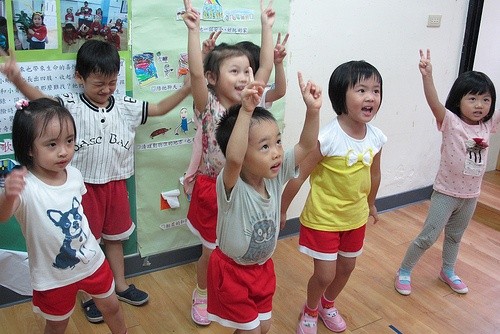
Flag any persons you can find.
[0,39,192,323]
[0,97,130,334]
[28,12,48,50]
[278,60,387,334]
[207,66,323,334]
[184,0,290,325]
[63,1,124,50]
[394,48,500,295]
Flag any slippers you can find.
[116,284,149,305]
[81,298,103,322]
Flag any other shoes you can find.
[296,308,318,334]
[395,268,412,295]
[439,268,468,293]
[319,301,346,333]
[190,289,212,325]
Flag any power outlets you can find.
[426,14,442,27]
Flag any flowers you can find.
[466,137,489,163]
